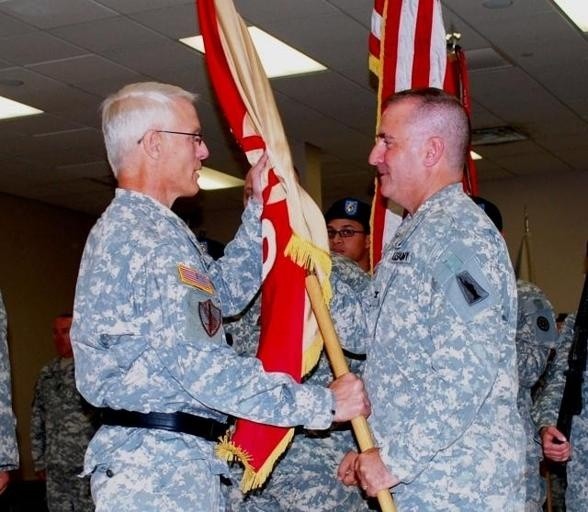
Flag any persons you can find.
[328,85,527,511]
[322,197,374,274]
[28,311,98,512]
[219,160,373,512]
[68,80,370,512]
[0,288,22,496]
[528,274,587,512]
[467,192,559,512]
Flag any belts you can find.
[295,420,351,436]
[101,405,228,443]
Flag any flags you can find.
[446,43,479,197]
[368,1,456,271]
[515,228,536,286]
[195,0,333,498]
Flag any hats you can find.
[471,196,502,232]
[325,197,370,228]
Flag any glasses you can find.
[327,228,367,239]
[138,126,205,150]
